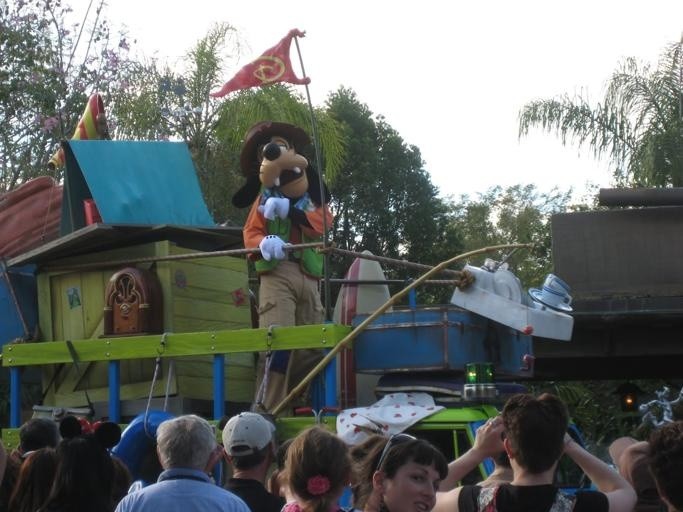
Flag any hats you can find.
[221,411,277,457]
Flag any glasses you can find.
[376,432,418,470]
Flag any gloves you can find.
[258,234,291,261]
[259,196,291,221]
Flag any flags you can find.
[208,28,311,98]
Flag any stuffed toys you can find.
[232,120,333,415]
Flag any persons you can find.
[476,435,514,485]
[279,426,351,512]
[11,418,58,466]
[221,412,287,512]
[350,433,449,512]
[633,420,683,511]
[6,448,57,511]
[610,439,649,486]
[431,392,637,512]
[114,414,252,512]
[35,416,121,512]
